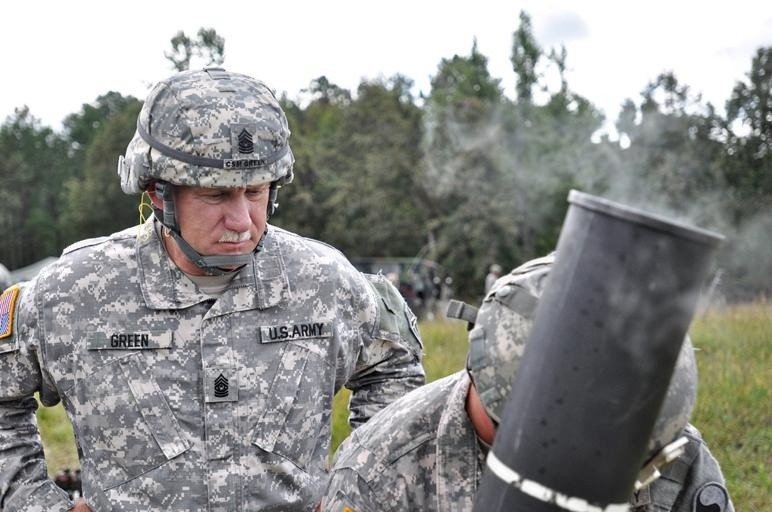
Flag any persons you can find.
[484,263,503,295]
[0,67,427,512]
[320,249,736,512]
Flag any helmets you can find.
[117,65,296,195]
[463,246,699,469]
